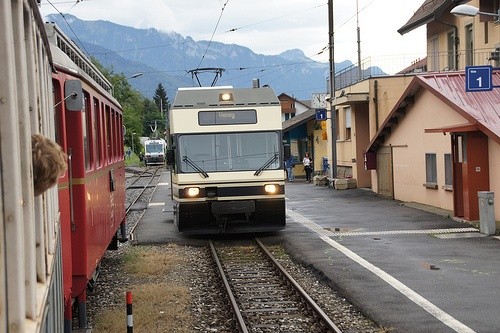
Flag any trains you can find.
[0,0,125,333]
[138,137,167,166]
[164,79,286,236]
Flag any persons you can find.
[139,152,145,162]
[285,155,295,183]
[302,152,314,183]
[31,133,68,198]
